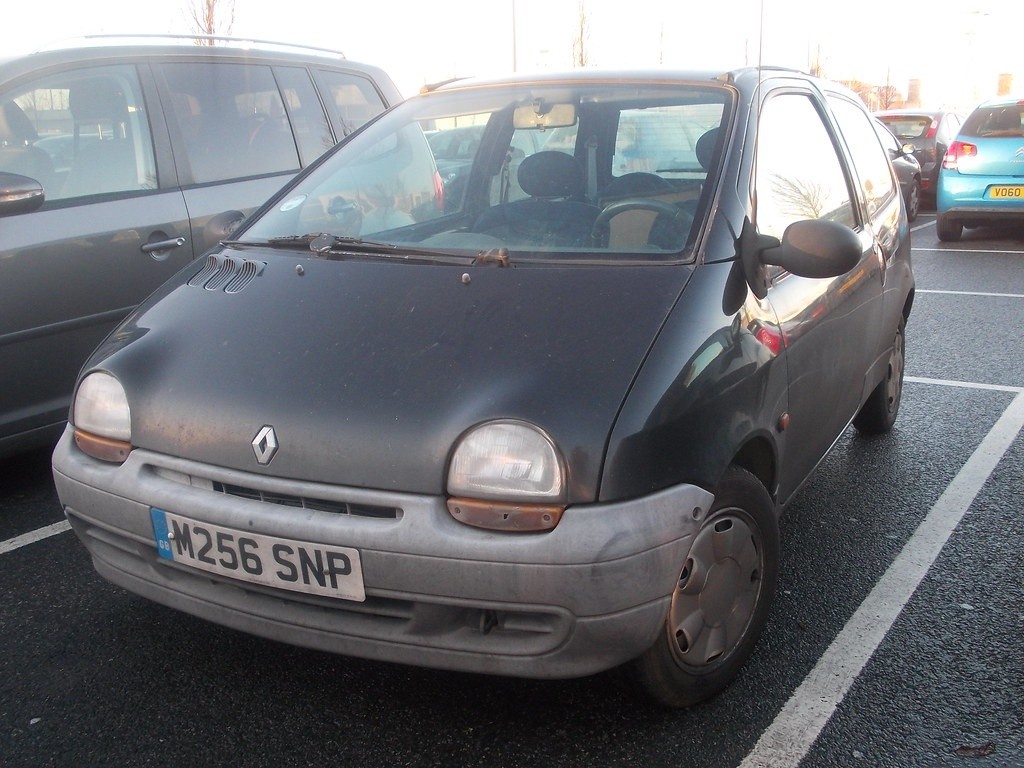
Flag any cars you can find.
[50,66,916,712]
[417,107,962,223]
[936,100,1024,242]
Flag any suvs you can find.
[1,35,444,470]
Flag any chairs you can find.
[643,125,721,250]
[63,75,135,193]
[468,150,611,249]
[0,95,54,187]
